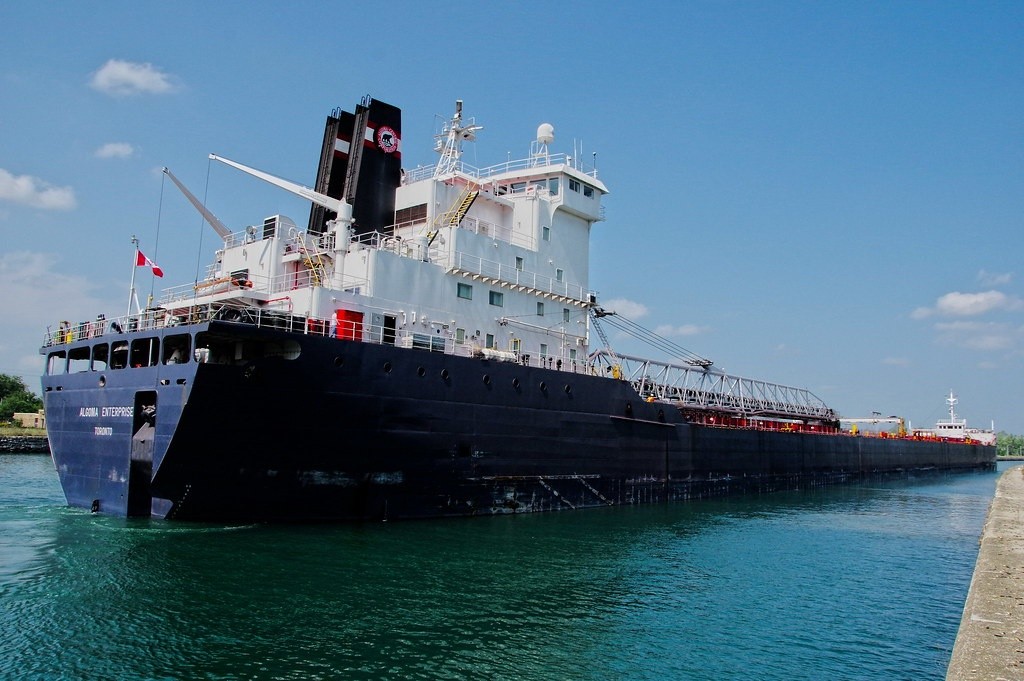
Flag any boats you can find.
[34,92,1000,527]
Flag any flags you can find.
[135,251,163,277]
